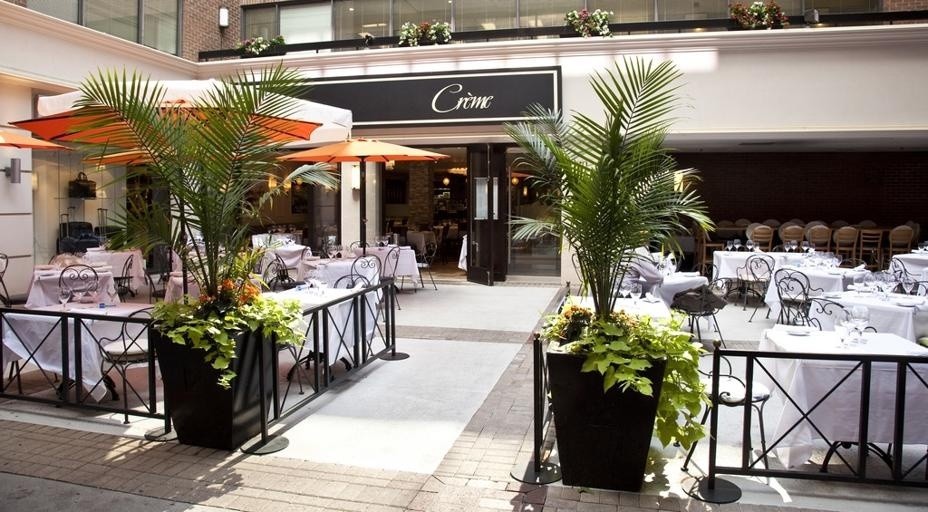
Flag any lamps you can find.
[219,4,229,49]
[3,157,21,184]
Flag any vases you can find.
[419,37,435,45]
[727,19,741,31]
[240,50,267,59]
[755,25,767,30]
[437,39,450,45]
[560,24,580,37]
[771,24,785,29]
[401,39,409,47]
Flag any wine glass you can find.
[727,241,732,251]
[802,241,809,252]
[839,308,856,336]
[277,233,296,247]
[88,280,103,305]
[809,244,816,253]
[918,243,924,255]
[659,259,667,271]
[746,240,753,252]
[326,245,346,263]
[833,255,842,269]
[852,305,869,344]
[71,280,87,307]
[619,280,630,303]
[791,240,798,252]
[303,270,328,296]
[924,241,928,250]
[56,287,71,311]
[903,278,914,294]
[833,319,851,350]
[784,242,791,253]
[630,285,641,306]
[382,236,389,247]
[734,240,741,251]
[375,236,381,248]
[854,271,898,300]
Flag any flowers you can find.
[235,35,268,54]
[747,2,769,26]
[414,23,437,41]
[563,9,614,37]
[765,0,788,25]
[397,22,418,47]
[727,1,748,24]
[428,21,453,42]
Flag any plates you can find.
[897,297,924,307]
[822,291,842,298]
[786,327,812,336]
[854,268,865,272]
[829,271,846,274]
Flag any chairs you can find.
[782,225,804,252]
[888,225,913,261]
[265,275,296,293]
[673,352,771,487]
[400,241,437,294]
[351,254,387,322]
[763,219,780,226]
[262,258,278,292]
[350,241,370,251]
[833,226,859,266]
[423,231,436,244]
[906,221,920,246]
[802,297,850,332]
[142,267,166,303]
[716,220,734,238]
[383,246,401,311]
[831,220,849,229]
[892,282,927,297]
[735,219,751,235]
[735,253,776,311]
[751,225,773,250]
[774,268,824,326]
[790,219,804,226]
[333,274,371,290]
[807,224,832,252]
[860,229,883,270]
[272,253,305,291]
[748,257,774,321]
[885,258,919,283]
[99,306,167,424]
[691,262,719,283]
[0,252,13,308]
[858,220,877,229]
[114,254,135,302]
[695,225,724,276]
[672,277,733,350]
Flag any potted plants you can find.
[267,36,287,56]
[499,54,715,493]
[59,56,340,452]
